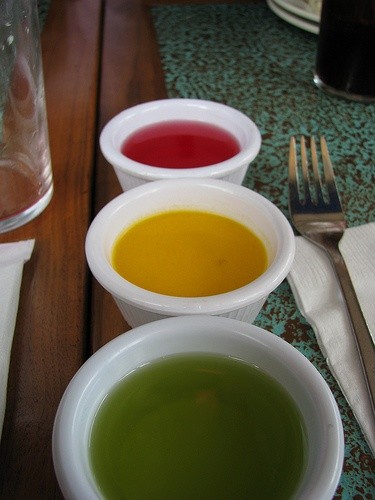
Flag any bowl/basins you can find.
[86,179,294,328]
[50,315,345,498]
[99,97,259,190]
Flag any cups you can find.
[314,1,373,103]
[1,0,54,233]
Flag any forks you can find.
[286,134,373,402]
